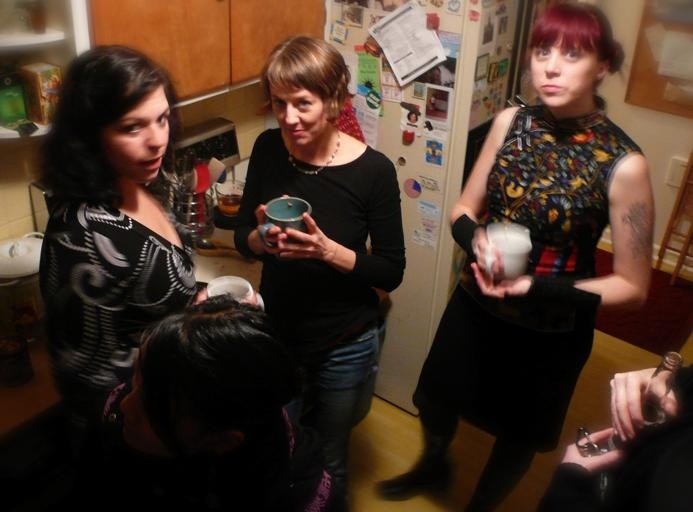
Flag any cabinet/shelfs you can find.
[0,0,74,138]
[84,0,327,111]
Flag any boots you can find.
[375,411,459,501]
[466,437,535,512]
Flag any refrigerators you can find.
[322,0,537,415]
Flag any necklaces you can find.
[286,131,341,176]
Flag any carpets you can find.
[590,246,692,362]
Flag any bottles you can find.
[608,346,681,456]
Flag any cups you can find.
[481,222,533,281]
[213,181,244,213]
[204,192,214,237]
[258,197,312,249]
[206,274,267,314]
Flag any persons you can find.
[76,295,340,511]
[378,1,654,511]
[232,34,408,512]
[534,348,691,511]
[39,44,258,410]
